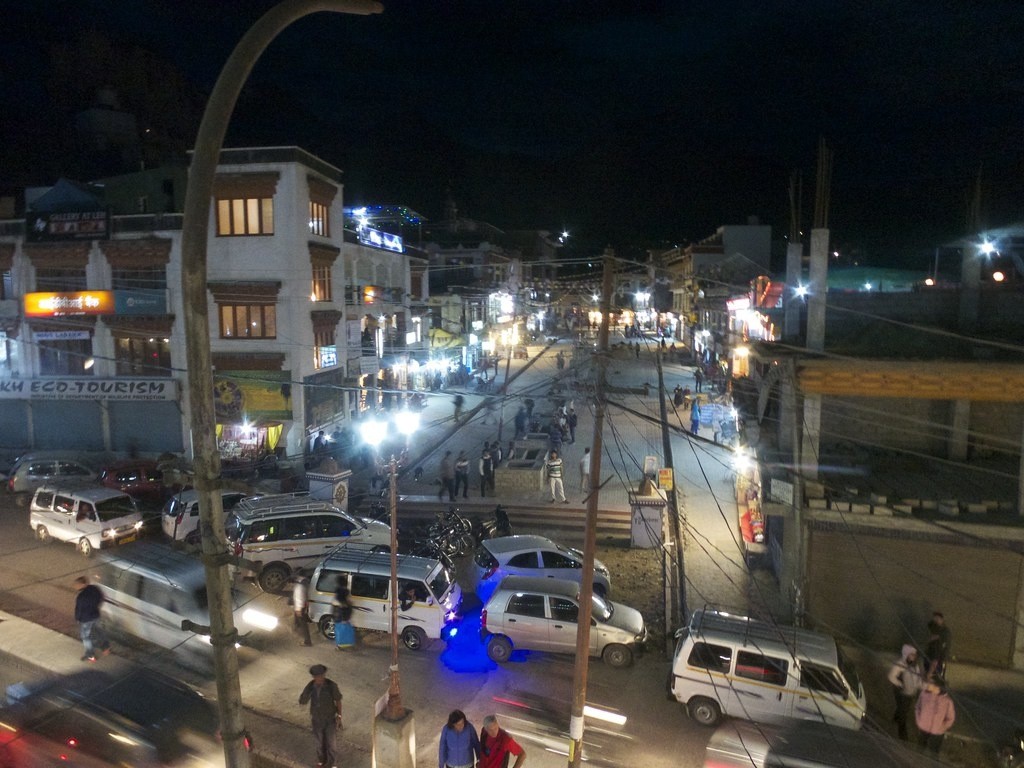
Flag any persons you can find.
[439,709,526,768]
[557,316,703,411]
[886,644,924,741]
[299,664,344,768]
[546,450,570,504]
[292,566,312,646]
[549,424,566,458]
[398,583,418,612]
[329,575,352,621]
[923,611,951,680]
[312,326,534,522]
[73,576,112,664]
[690,396,701,435]
[914,676,956,761]
[579,447,590,494]
[334,609,354,652]
[567,409,577,444]
[557,409,567,427]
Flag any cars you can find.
[0,657,254,768]
[6,453,101,506]
[26,478,145,562]
[162,487,251,548]
[94,459,164,501]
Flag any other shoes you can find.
[103,642,112,654]
[81,655,97,662]
[562,500,570,503]
[552,499,556,504]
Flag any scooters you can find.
[406,504,516,576]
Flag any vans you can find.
[304,543,463,651]
[666,608,867,730]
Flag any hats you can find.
[310,664,326,675]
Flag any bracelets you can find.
[338,714,341,717]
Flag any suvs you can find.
[220,490,401,593]
[479,574,648,667]
[468,533,613,601]
[90,541,274,675]
